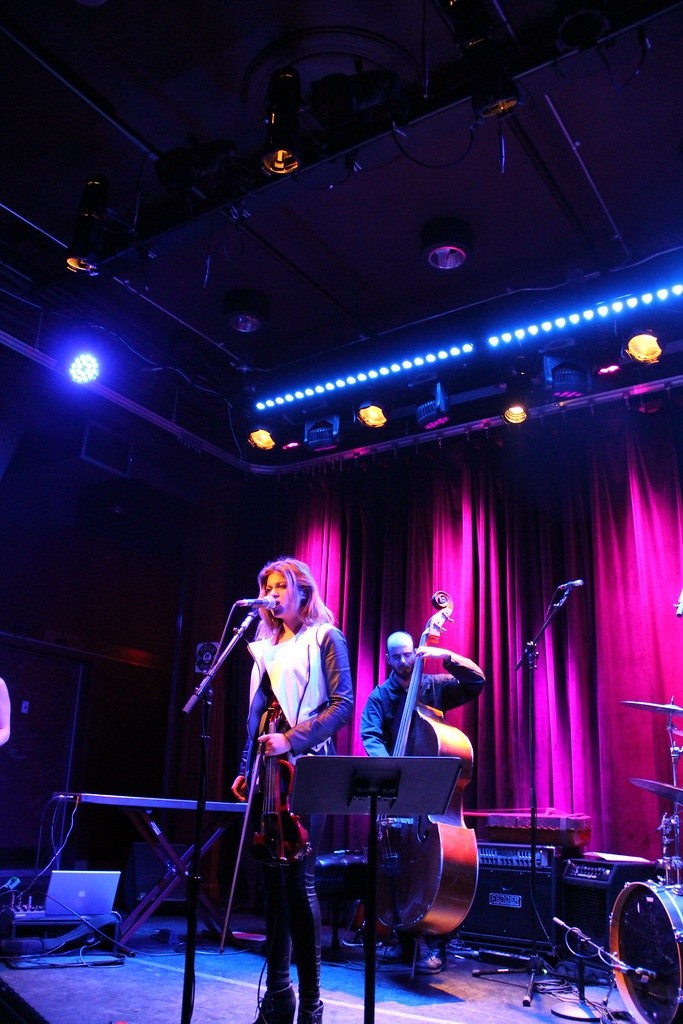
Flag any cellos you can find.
[359,589,482,984]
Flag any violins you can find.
[248,699,313,868]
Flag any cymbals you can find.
[618,700,683,717]
[628,776,683,805]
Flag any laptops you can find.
[44,870,122,917]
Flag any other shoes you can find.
[377,944,406,963]
[415,948,448,974]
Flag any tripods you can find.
[471,594,611,1009]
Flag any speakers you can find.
[459,843,584,957]
[563,857,665,971]
[124,842,193,915]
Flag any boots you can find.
[249,984,297,1024]
[296,999,324,1024]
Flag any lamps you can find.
[466,36,525,120]
[64,188,105,277]
[356,396,390,430]
[420,218,473,271]
[552,355,586,397]
[248,420,283,452]
[303,412,341,453]
[223,287,271,334]
[500,368,531,425]
[259,65,303,179]
[620,318,664,366]
[415,380,451,431]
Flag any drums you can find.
[606,879,683,1024]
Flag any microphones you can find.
[633,986,667,1004]
[558,580,584,590]
[235,595,276,610]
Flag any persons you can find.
[360,632,486,757]
[230,559,354,1024]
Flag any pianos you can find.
[53,792,248,956]
